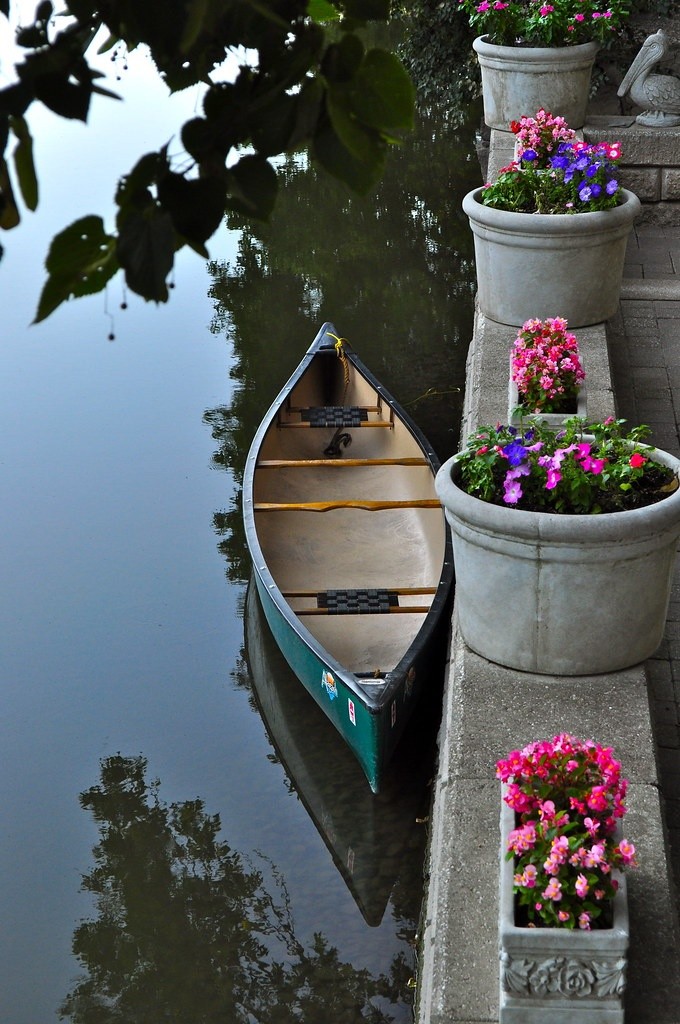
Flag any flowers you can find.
[458,0,626,43]
[497,731,642,933]
[481,105,624,212]
[456,401,669,513]
[510,315,585,415]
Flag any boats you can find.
[245,568,445,928]
[242,322,455,796]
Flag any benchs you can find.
[300,406,368,429]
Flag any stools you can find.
[316,589,400,615]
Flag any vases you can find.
[498,766,634,1024]
[436,431,680,676]
[504,352,589,431]
[473,34,606,131]
[462,177,642,330]
